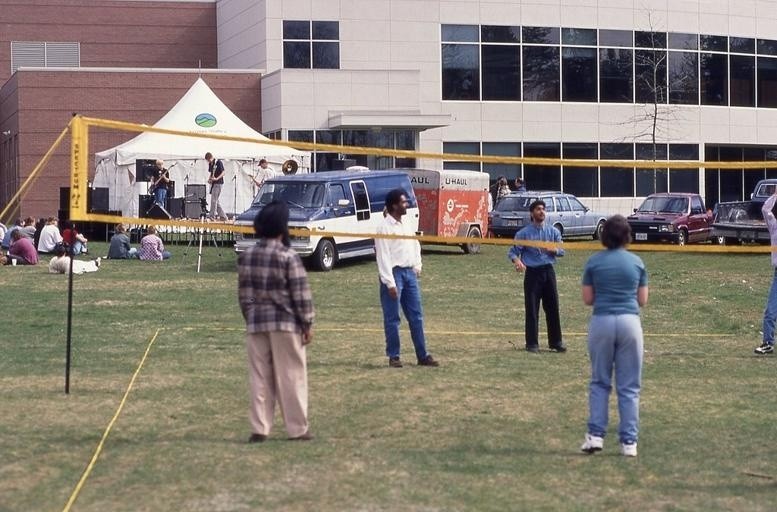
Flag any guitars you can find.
[148,161,179,194]
[209,165,217,194]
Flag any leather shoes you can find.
[524,342,541,352]
[548,341,567,351]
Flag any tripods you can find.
[179,212,223,272]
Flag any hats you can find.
[258,159,267,166]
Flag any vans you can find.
[231,167,422,273]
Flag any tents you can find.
[93,78,312,215]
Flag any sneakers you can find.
[290,431,316,444]
[418,355,440,366]
[247,432,267,443]
[580,432,604,455]
[755,341,776,354]
[619,441,639,457]
[387,355,404,369]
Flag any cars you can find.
[488,188,609,240]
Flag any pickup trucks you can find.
[620,189,726,245]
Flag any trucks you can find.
[709,176,777,244]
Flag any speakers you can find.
[89,187,109,210]
[136,158,160,182]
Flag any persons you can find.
[256,159,276,202]
[102,223,137,259]
[490,175,526,207]
[754,185,777,354]
[151,159,169,216]
[0,216,101,276]
[581,214,649,457]
[135,226,172,260]
[205,152,229,222]
[374,189,442,368]
[508,200,566,354]
[237,203,315,442]
